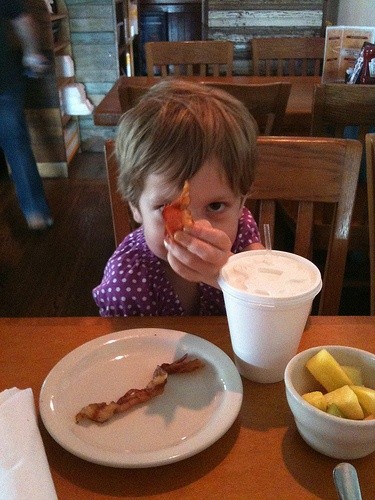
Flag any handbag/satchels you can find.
[346,42,375,85]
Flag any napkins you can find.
[0,386,59,499]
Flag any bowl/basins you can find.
[283,345,375,460]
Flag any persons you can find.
[0,0,54,232]
[92,75,266,318]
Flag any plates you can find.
[38,328,243,469]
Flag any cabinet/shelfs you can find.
[5,0,83,180]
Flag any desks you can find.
[92,74,343,128]
[0,315,375,499]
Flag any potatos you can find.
[300,349,375,419]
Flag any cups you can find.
[217,250,322,384]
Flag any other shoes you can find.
[28,216,54,230]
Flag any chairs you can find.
[105,80,375,314]
[250,37,322,77]
[143,40,234,78]
